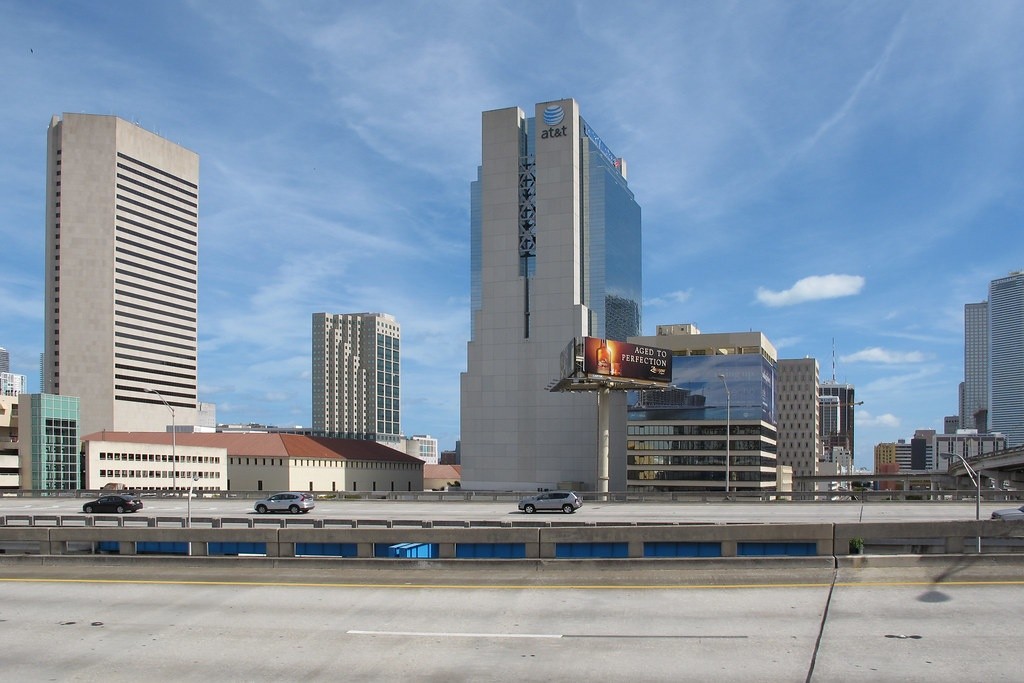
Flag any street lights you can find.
[939,452,982,554]
[142,385,176,492]
[186,473,201,557]
[718,373,731,496]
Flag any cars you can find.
[253,491,316,514]
[83,493,144,513]
[518,490,582,514]
